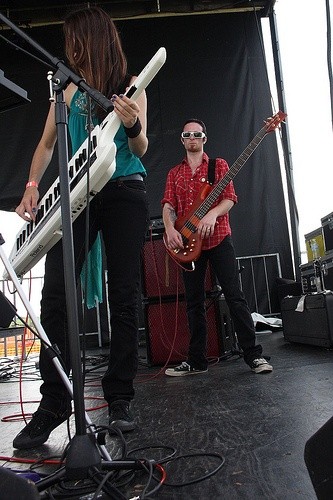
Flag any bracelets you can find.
[122,117,142,139]
[25,182,39,188]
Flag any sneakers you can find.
[108,400,136,432]
[164,362,208,376]
[13,403,72,449]
[251,357,273,373]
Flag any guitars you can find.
[162,107,289,265]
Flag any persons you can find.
[165,119,272,376]
[13,6,148,446]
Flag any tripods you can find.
[0,14,155,500]
[207,321,271,362]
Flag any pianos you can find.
[1,45,168,284]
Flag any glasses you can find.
[181,131,206,139]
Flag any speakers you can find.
[0,291,17,328]
[0,466,41,500]
[142,232,241,367]
[304,417,333,500]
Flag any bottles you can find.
[312,239,320,260]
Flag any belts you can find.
[109,174,144,182]
